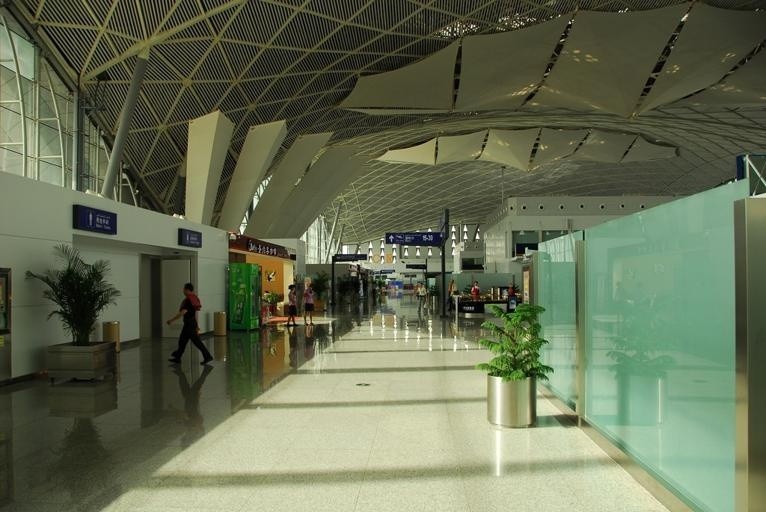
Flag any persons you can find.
[470,281,481,301]
[302,324,315,362]
[303,286,315,326]
[285,284,298,327]
[167,363,215,438]
[286,326,297,368]
[166,282,213,366]
[414,280,460,312]
[415,309,430,336]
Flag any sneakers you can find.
[304,323,314,325]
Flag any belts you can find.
[419,295,425,297]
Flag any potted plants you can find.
[605,303,679,426]
[309,272,330,312]
[24,240,123,387]
[475,303,554,428]
[24,379,123,512]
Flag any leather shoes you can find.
[287,323,297,326]
[168,358,181,362]
[200,356,213,364]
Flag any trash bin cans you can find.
[214,312,227,336]
[103,322,120,353]
[429,290,439,316]
